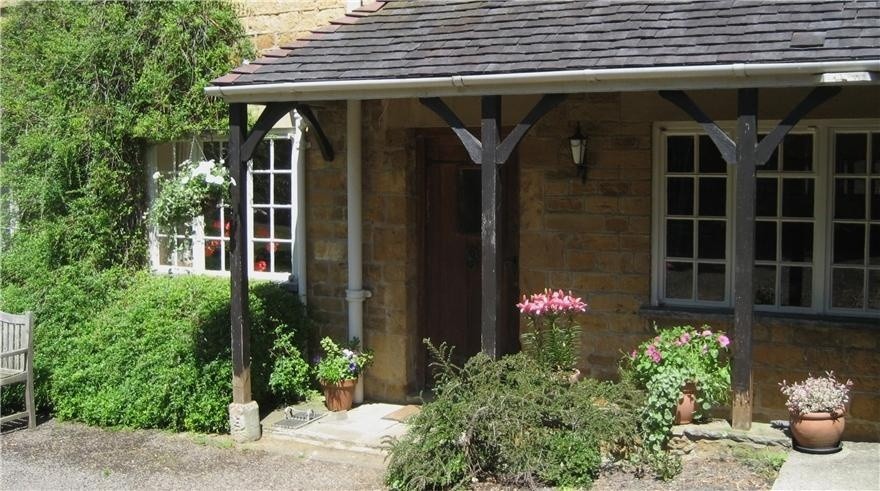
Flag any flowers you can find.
[311,337,375,387]
[515,285,587,377]
[778,370,855,423]
[144,156,237,259]
[618,319,734,420]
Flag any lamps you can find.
[569,119,589,185]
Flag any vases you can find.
[673,382,700,425]
[320,377,358,413]
[788,409,846,455]
[547,370,581,410]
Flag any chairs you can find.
[1,309,37,431]
